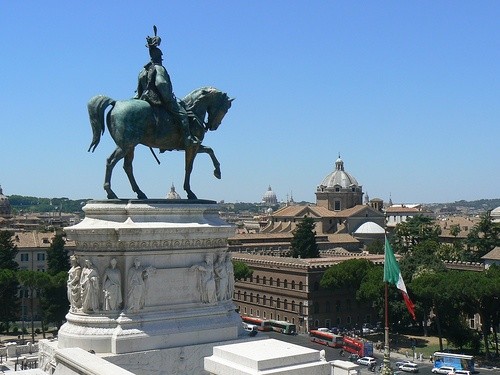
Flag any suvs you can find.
[432,366,455,375]
[447,369,471,375]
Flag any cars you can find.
[348,353,360,362]
[317,321,385,337]
[395,360,414,367]
[357,356,377,366]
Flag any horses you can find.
[87,86,236,200]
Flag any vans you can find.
[398,362,419,373]
[243,323,257,336]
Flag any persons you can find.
[369,363,374,369]
[194,253,218,306]
[126,258,148,315]
[101,256,125,313]
[412,351,418,362]
[379,363,383,372]
[308,322,384,354]
[280,329,297,336]
[216,253,236,303]
[131,25,203,144]
[65,253,103,315]
[428,352,435,365]
[417,352,425,363]
[405,350,409,360]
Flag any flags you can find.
[383,234,416,319]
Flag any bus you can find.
[239,315,272,332]
[269,319,296,335]
[342,334,373,358]
[308,329,344,349]
[432,351,474,374]
